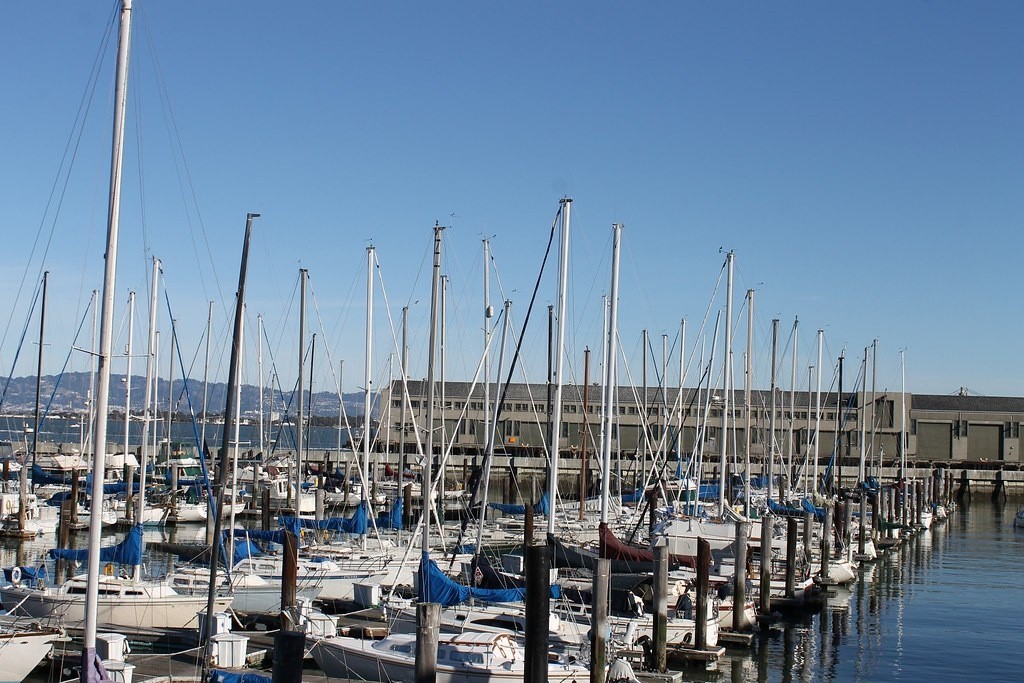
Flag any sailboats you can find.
[0,1,961,683]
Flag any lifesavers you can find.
[6,499,11,509]
[103,563,113,577]
[12,566,22,583]
